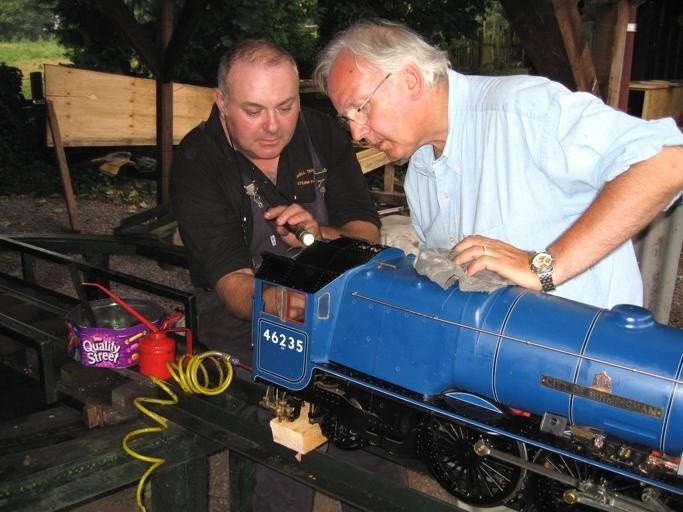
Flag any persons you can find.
[319,21,683,321]
[169,36,400,511]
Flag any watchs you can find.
[527,244,556,294]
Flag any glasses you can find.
[338,72,395,134]
